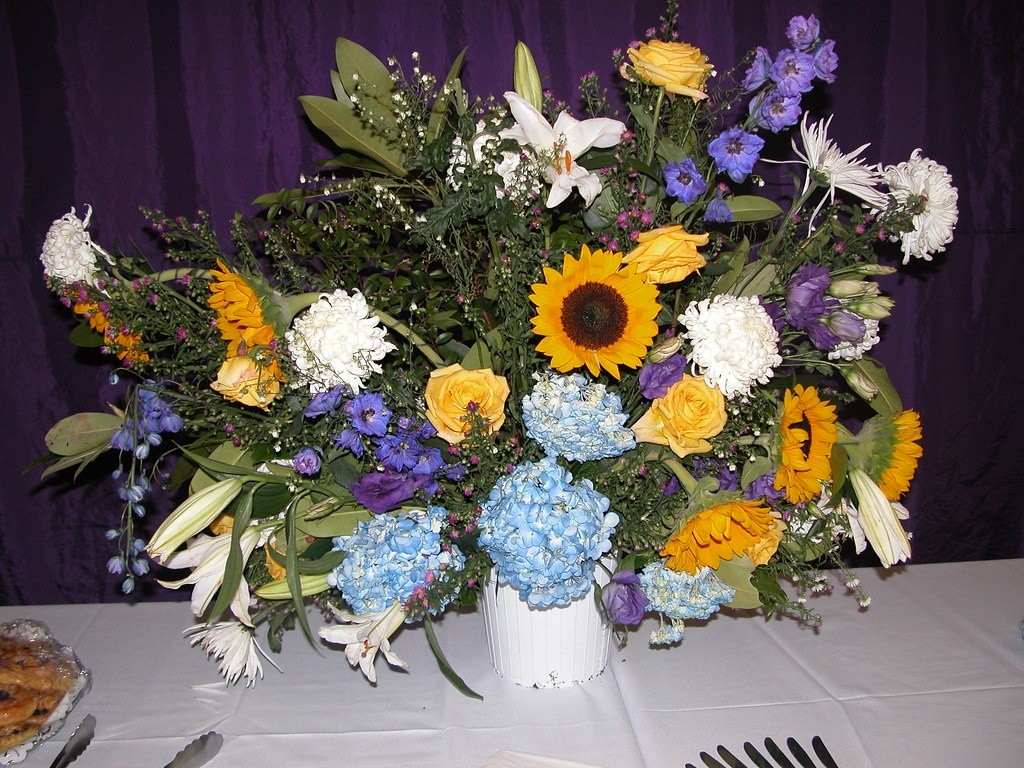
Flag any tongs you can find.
[50,713,224,768]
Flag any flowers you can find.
[41,0,955,699]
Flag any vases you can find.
[479,551,618,688]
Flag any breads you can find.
[0,634,76,755]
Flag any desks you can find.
[0,561,1024,767]
